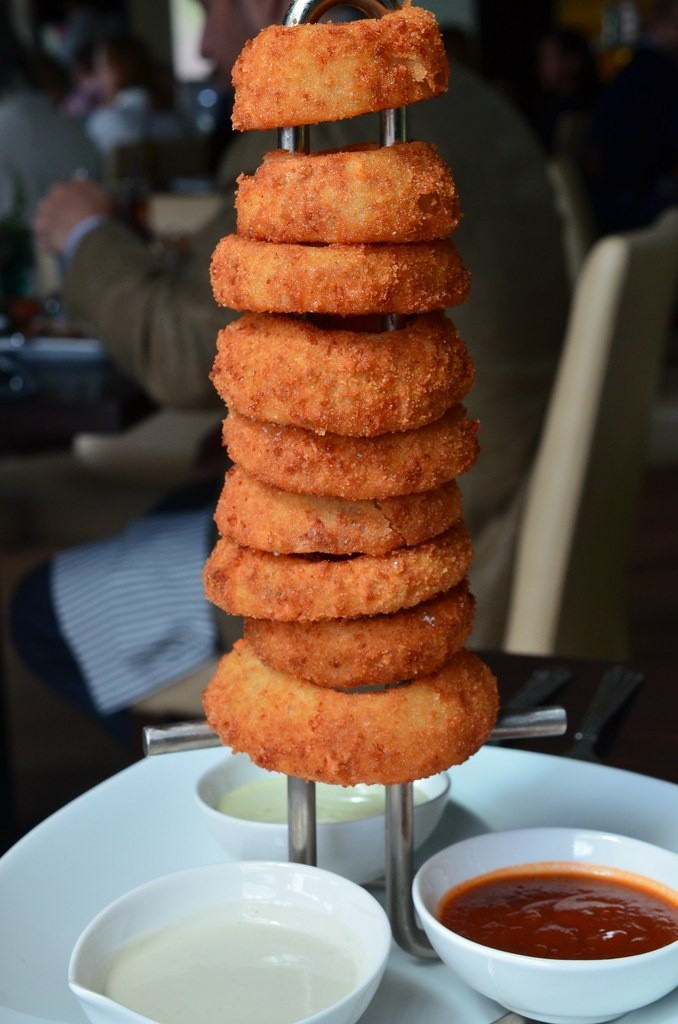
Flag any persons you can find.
[1,0,677,761]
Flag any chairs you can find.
[502,209,678,662]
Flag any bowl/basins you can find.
[410,827,678,1024]
[194,752,451,884]
[67,859,392,1024]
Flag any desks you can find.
[0,746,678,1024]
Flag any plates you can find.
[0,746,678,1024]
